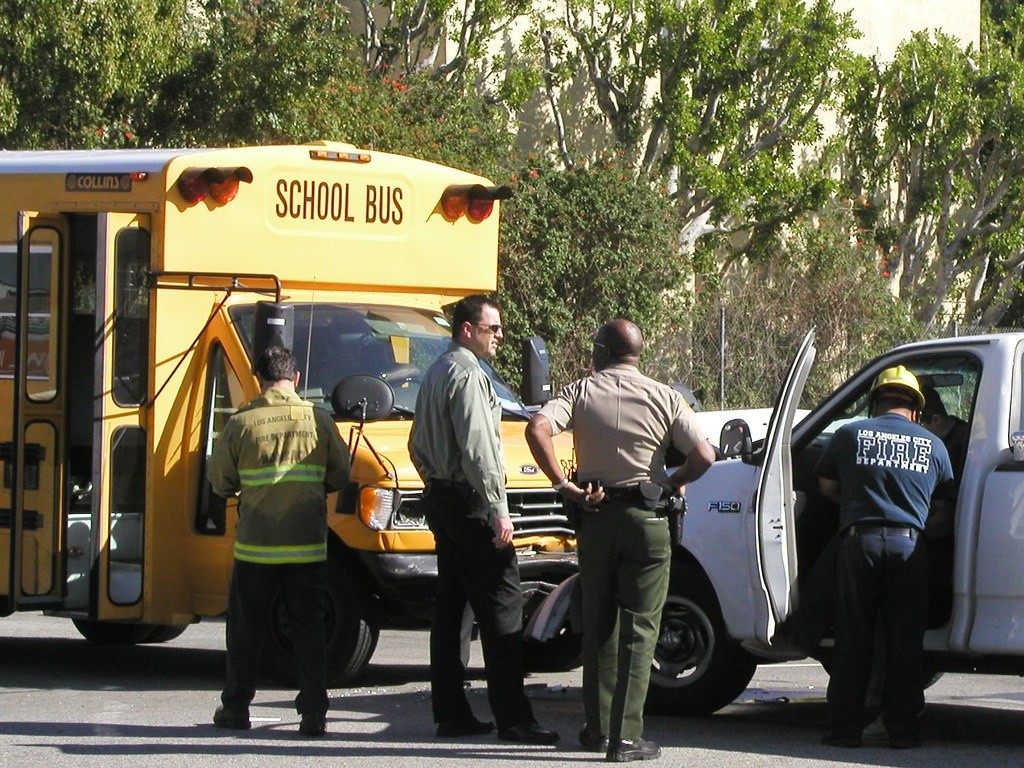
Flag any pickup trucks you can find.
[644,325,1024,716]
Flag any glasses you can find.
[592,340,606,348]
[473,322,504,334]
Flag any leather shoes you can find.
[498,719,560,744]
[437,715,496,737]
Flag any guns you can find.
[666,493,685,546]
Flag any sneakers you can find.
[606,737,658,761]
[579,723,609,752]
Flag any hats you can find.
[924,387,946,411]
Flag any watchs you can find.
[552,478,568,490]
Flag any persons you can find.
[407,294,561,744]
[817,365,966,749]
[207,345,351,735]
[524,319,715,763]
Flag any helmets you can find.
[868,365,925,410]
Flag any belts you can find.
[843,524,924,542]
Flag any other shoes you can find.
[890,733,922,749]
[214,707,252,731]
[823,729,863,747]
[298,710,325,734]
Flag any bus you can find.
[0,140,580,684]
